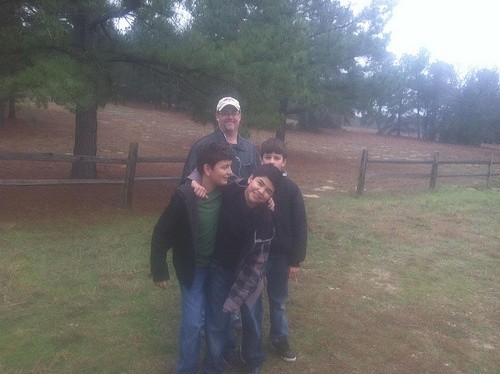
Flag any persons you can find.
[180,97,307,374]
[151,142,275,374]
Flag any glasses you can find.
[218,110,241,116]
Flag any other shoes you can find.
[270,341,297,361]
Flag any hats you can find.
[216,96,241,112]
